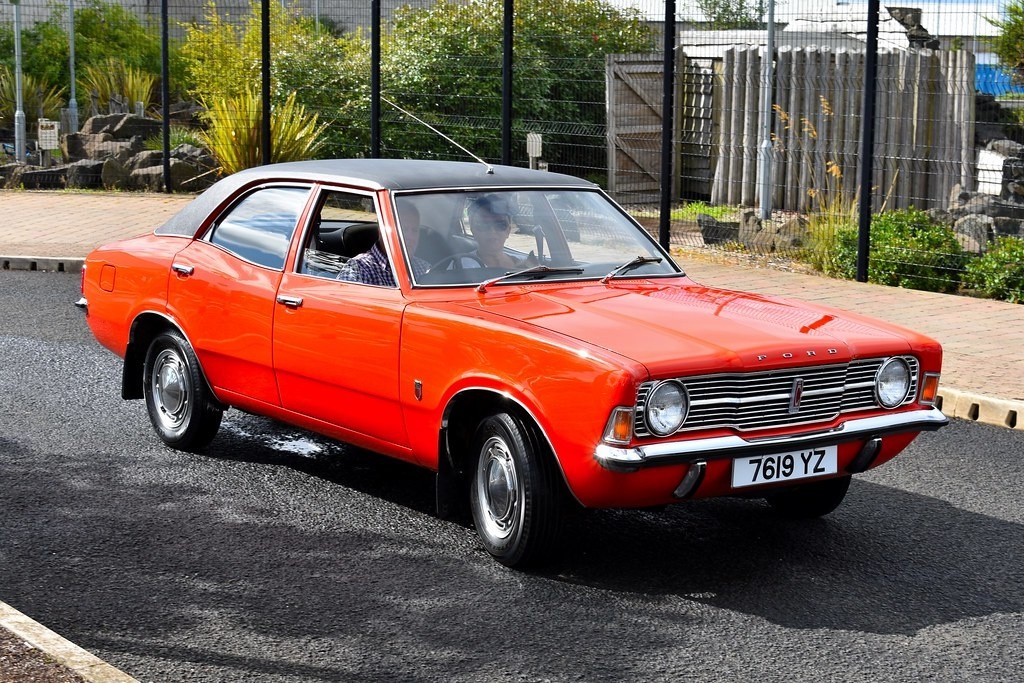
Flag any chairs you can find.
[341,220,452,268]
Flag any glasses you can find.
[470,220,509,232]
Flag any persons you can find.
[446,194,528,270]
[336,199,433,289]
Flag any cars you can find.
[75,156,949,570]
